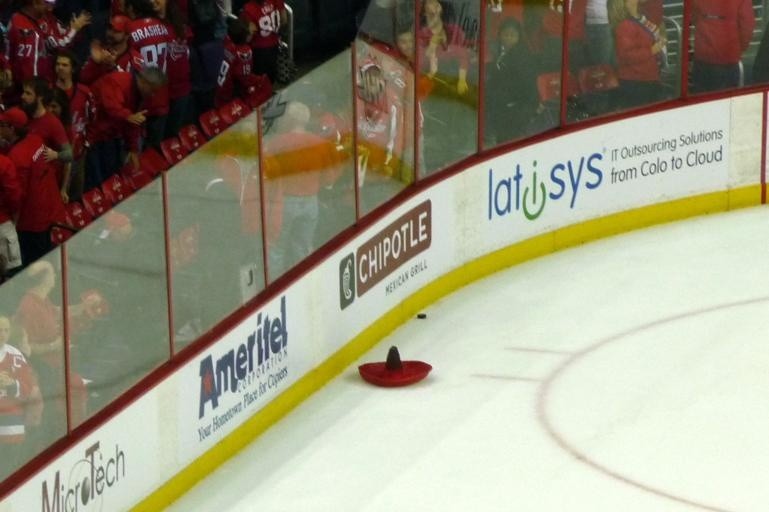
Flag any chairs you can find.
[43,98,252,254]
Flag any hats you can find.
[109,16,135,32]
[0,106,28,129]
